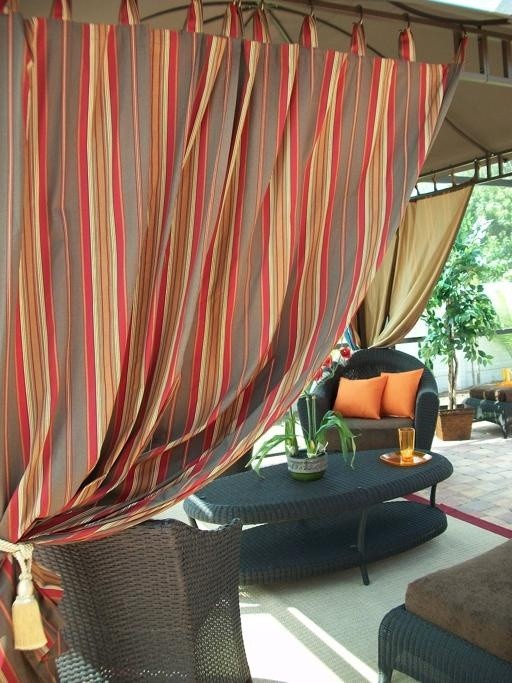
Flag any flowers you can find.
[245,346,357,478]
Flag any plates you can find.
[380,450,432,467]
[496,382,512,388]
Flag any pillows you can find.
[332,374,389,422]
[382,367,424,417]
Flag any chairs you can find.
[28,519,253,683]
[297,348,439,452]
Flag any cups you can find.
[398,427,415,460]
[502,368,512,381]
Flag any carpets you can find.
[240,491,512,683]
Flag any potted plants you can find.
[417,240,502,441]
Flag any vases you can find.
[288,449,328,480]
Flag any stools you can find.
[462,385,512,439]
[378,538,512,683]
